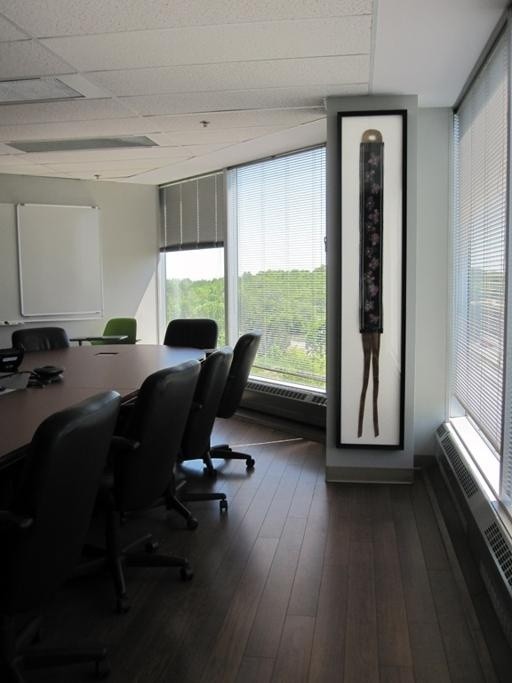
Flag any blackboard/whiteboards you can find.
[16,203,101,317]
[0,203,104,326]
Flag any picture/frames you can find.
[337,109,407,451]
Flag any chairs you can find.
[143,349,230,531]
[186,333,263,478]
[161,319,221,349]
[8,325,71,353]
[73,360,201,614]
[3,386,122,682]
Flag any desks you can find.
[3,344,228,466]
